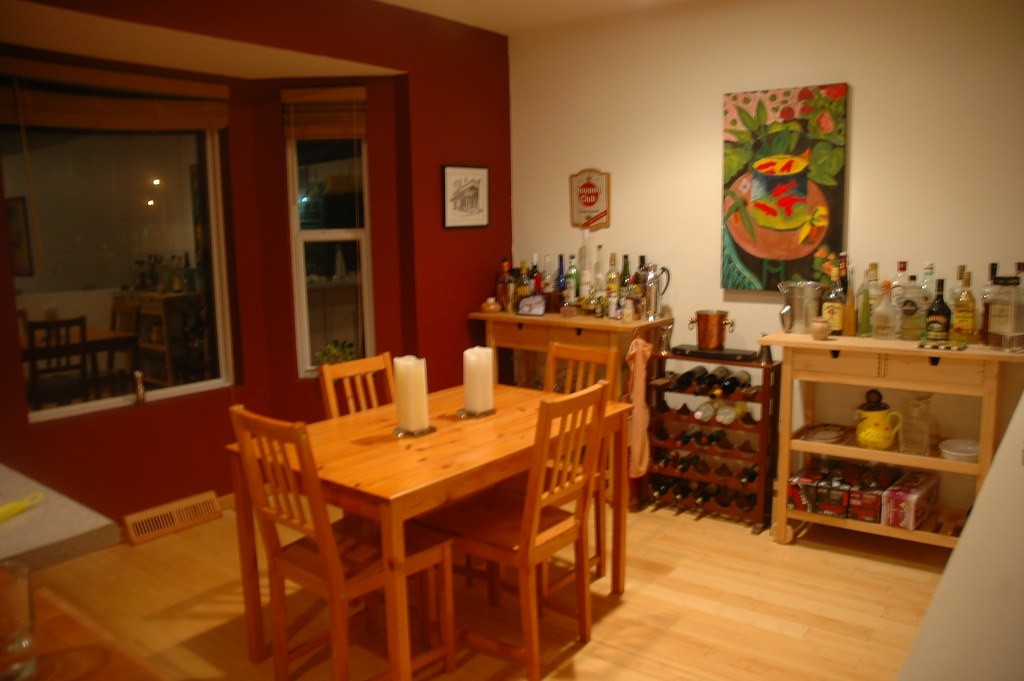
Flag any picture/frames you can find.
[441,163,491,229]
[6,196,33,275]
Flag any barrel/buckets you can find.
[780,281,820,334]
[687,310,735,350]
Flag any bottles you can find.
[133,249,195,293]
[896,391,941,457]
[652,366,760,507]
[480,227,646,322]
[820,252,1024,354]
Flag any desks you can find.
[467,309,675,400]
[16,327,137,399]
[222,380,634,680]
[0,461,124,681]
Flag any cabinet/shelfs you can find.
[113,290,196,387]
[639,348,783,528]
[756,329,1024,552]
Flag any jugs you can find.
[854,389,902,450]
[643,263,670,319]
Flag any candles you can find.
[462,345,495,416]
[392,355,430,434]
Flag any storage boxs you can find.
[816,462,865,517]
[881,470,941,531]
[786,456,836,512]
[849,464,902,524]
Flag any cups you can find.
[0,561,38,681]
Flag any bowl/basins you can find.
[938,439,979,463]
[804,423,846,443]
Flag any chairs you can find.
[408,379,610,681]
[314,351,421,626]
[27,314,92,409]
[87,303,139,393]
[227,403,455,680]
[464,342,621,613]
